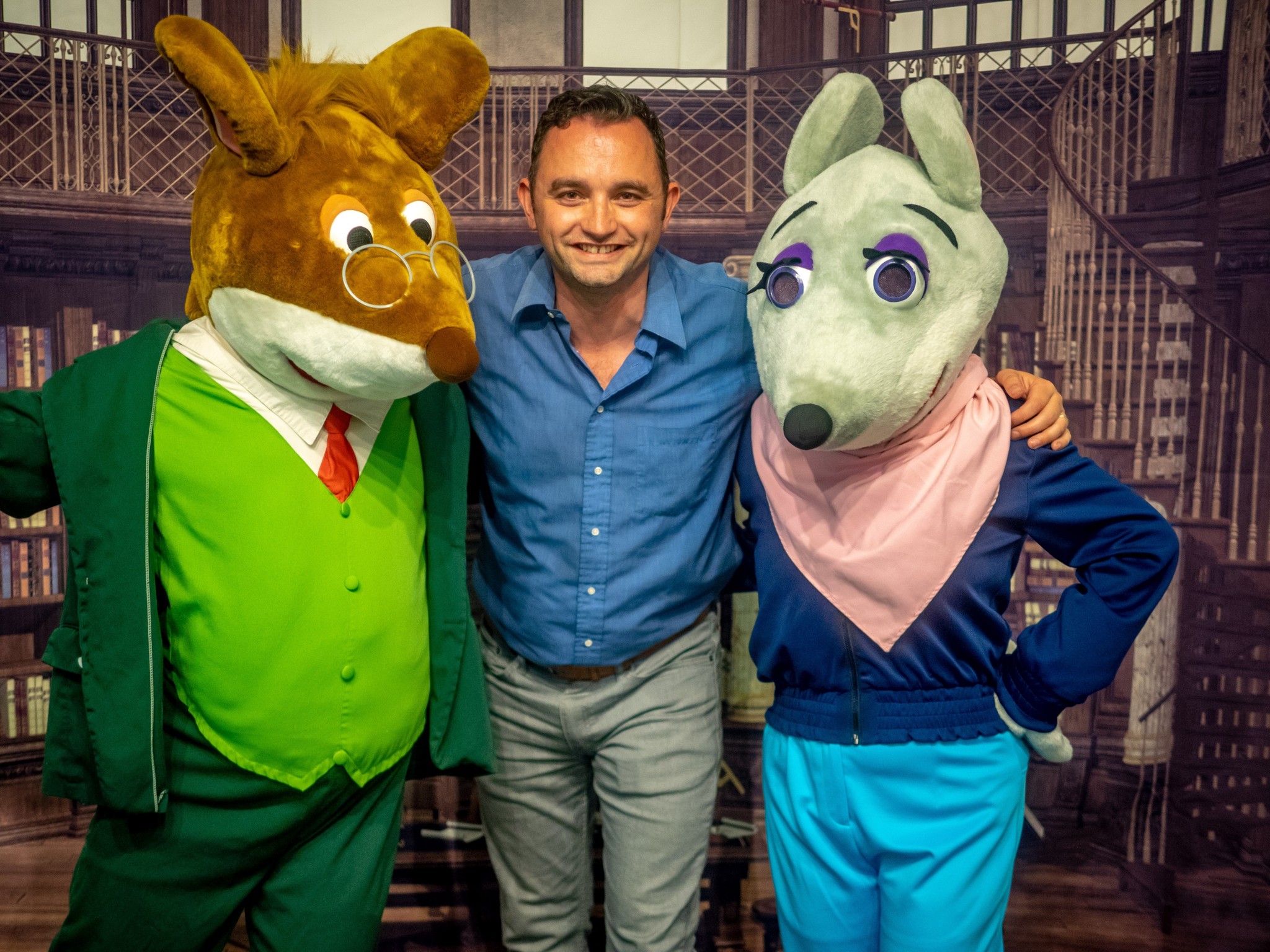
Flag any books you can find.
[0,537,58,599]
[0,674,51,738]
[0,307,137,387]
[0,505,60,529]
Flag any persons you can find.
[462,87,1072,952]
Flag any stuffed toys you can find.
[0,14,487,952]
[737,74,1179,952]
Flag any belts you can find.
[481,598,719,682]
